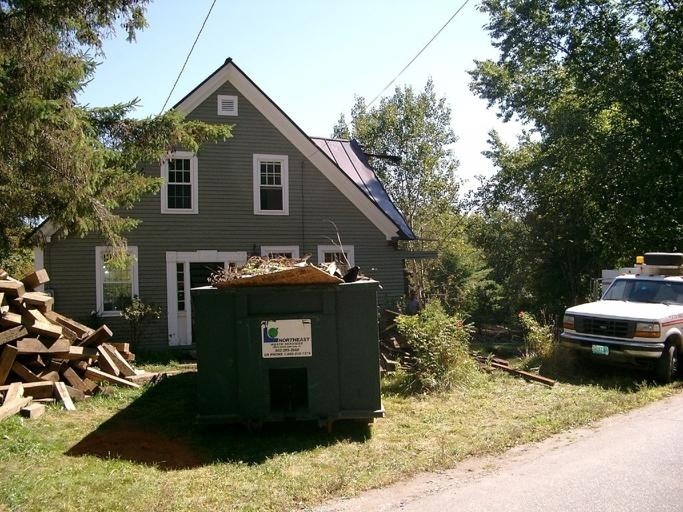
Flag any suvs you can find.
[558,253,682,384]
